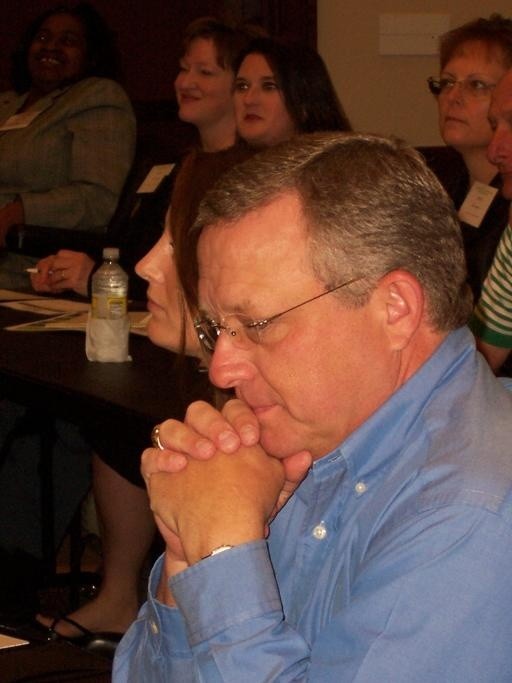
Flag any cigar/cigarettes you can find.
[24,268,55,275]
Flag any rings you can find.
[153,424,167,451]
[61,270,65,280]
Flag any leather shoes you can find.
[0,567,126,652]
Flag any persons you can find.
[109,129,512,683]
[29,34,354,651]
[0,2,139,291]
[1,13,272,614]
[468,67,512,376]
[410,18,512,302]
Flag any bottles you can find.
[85,246,133,363]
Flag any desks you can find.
[1,252,212,643]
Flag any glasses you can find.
[193,264,424,354]
[436,74,496,97]
